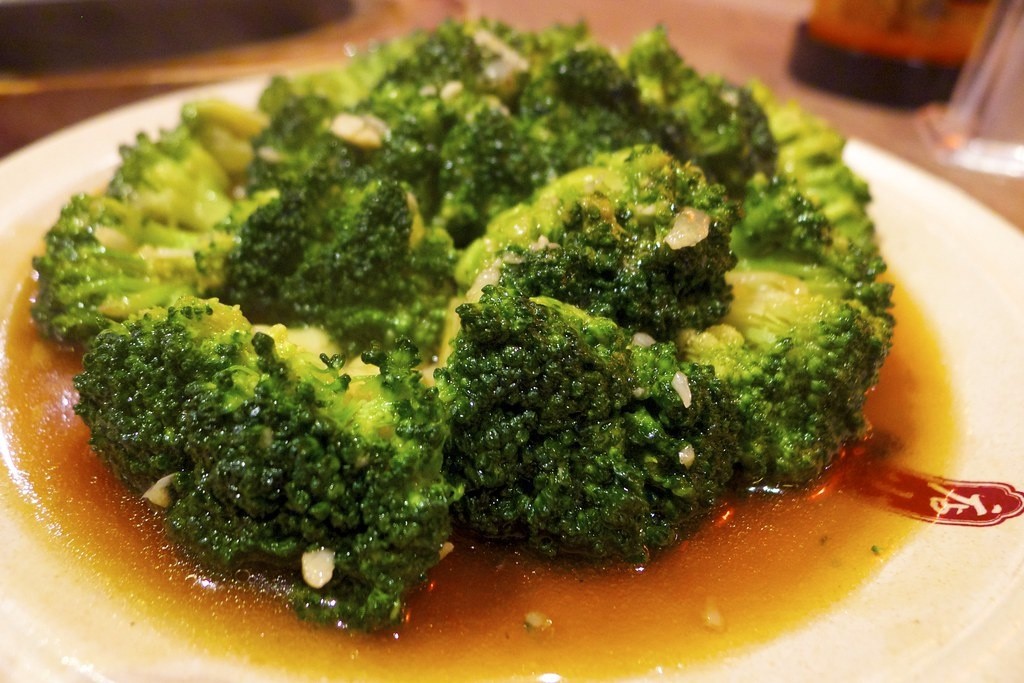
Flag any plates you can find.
[0,64,1022,683]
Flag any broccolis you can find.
[24,16,897,630]
